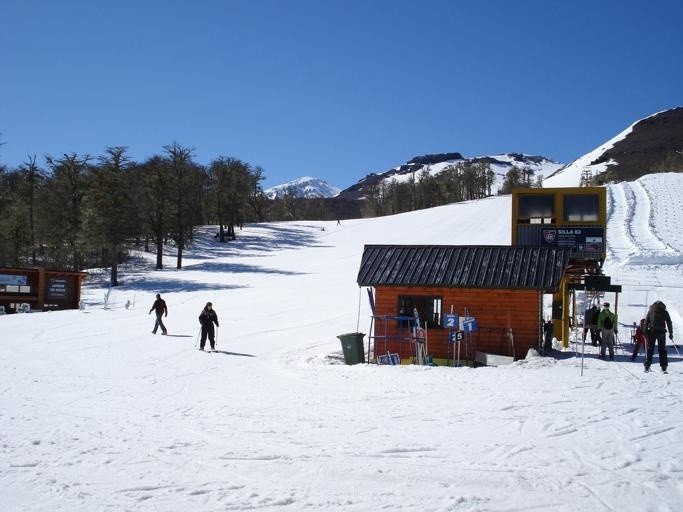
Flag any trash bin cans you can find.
[337,333,366,365]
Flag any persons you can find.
[632,319,645,359]
[199,302,219,350]
[644,301,673,371]
[150,294,167,335]
[582,306,602,347]
[598,302,618,361]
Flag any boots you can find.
[609,352,614,359]
[661,361,667,371]
[644,361,651,370]
[632,352,637,359]
[599,352,605,358]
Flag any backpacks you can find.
[604,316,613,330]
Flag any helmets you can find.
[640,319,646,325]
[603,302,609,307]
